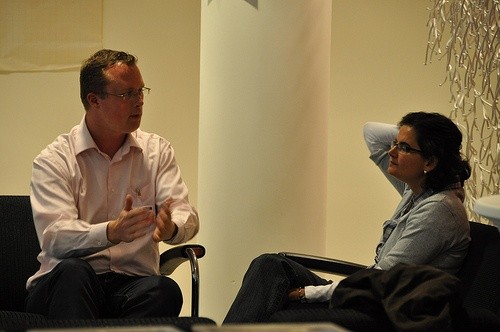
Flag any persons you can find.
[220,112,471,332]
[26,49,199,323]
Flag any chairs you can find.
[280,221,500,332]
[0,195,217,332]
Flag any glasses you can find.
[391,140,417,153]
[93,85,151,101]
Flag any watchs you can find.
[298,291,306,303]
